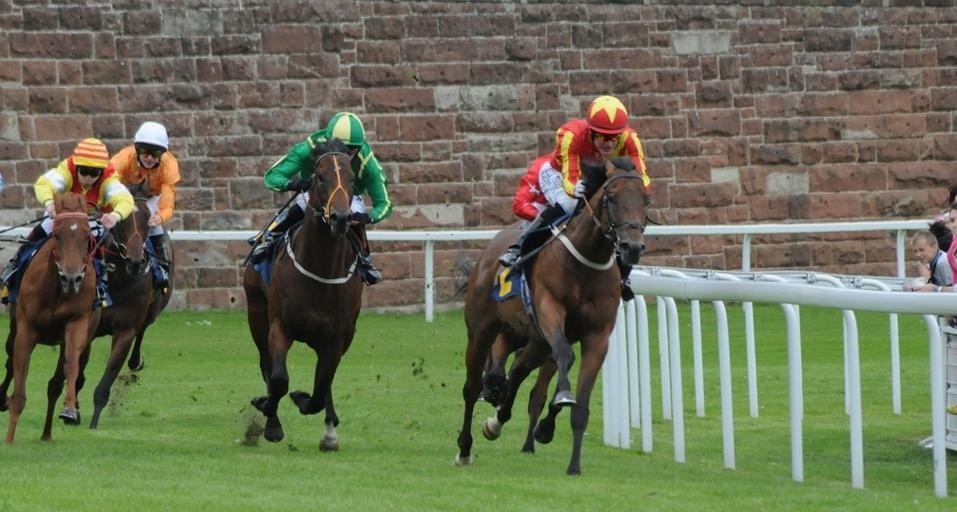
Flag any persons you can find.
[902,185,957,328]
[498,95,651,302]
[109,121,181,273]
[512,154,554,233]
[1,137,134,307]
[248,111,393,285]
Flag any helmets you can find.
[326,112,365,145]
[73,138,108,168]
[586,96,628,134]
[134,122,169,149]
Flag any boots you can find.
[0,224,49,287]
[248,205,304,246]
[499,213,546,267]
[150,235,168,270]
[358,263,378,284]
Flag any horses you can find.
[64,173,175,430]
[0,191,102,445]
[242,136,365,452]
[445,155,652,477]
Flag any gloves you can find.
[291,179,309,193]
[353,212,370,224]
[575,180,586,198]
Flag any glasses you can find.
[78,167,100,177]
[594,133,617,141]
[139,147,163,158]
